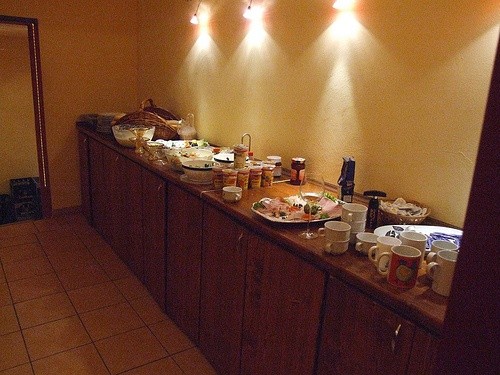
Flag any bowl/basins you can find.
[161,140,249,183]
[112,124,156,149]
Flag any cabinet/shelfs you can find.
[77,121,463,375]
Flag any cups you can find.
[323,203,368,255]
[221,186,243,203]
[355,232,379,255]
[376,245,421,290]
[426,240,458,265]
[426,250,459,296]
[399,231,427,269]
[368,236,402,268]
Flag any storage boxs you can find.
[9,177,42,224]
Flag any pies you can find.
[380,197,421,217]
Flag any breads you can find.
[167,119,181,130]
[110,115,122,125]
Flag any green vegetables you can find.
[315,192,339,219]
[253,201,267,209]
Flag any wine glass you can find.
[296,173,325,240]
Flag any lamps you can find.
[243,0,252,17]
[190,0,202,24]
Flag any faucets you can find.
[241,133,251,152]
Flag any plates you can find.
[374,225,463,254]
[251,197,347,223]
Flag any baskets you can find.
[378,198,431,224]
[115,98,180,140]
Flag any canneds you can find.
[267,156,282,178]
[290,158,306,185]
